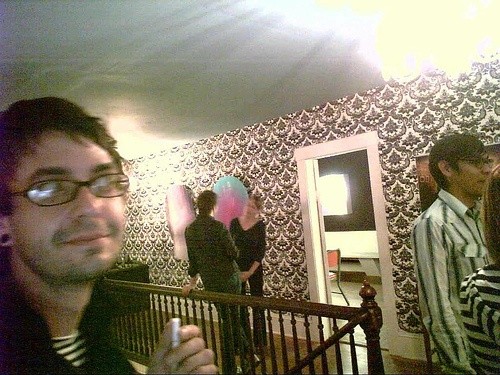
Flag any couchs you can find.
[101,262,150,317]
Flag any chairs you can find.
[327,250,350,306]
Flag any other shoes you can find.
[254,354,262,367]
[236,367,244,374]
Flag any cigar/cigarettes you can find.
[172,318,180,349]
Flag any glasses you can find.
[458,155,495,168]
[8,172,130,204]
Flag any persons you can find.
[181,190,260,375]
[1,98,217,375]
[228,193,267,355]
[410,133,500,375]
